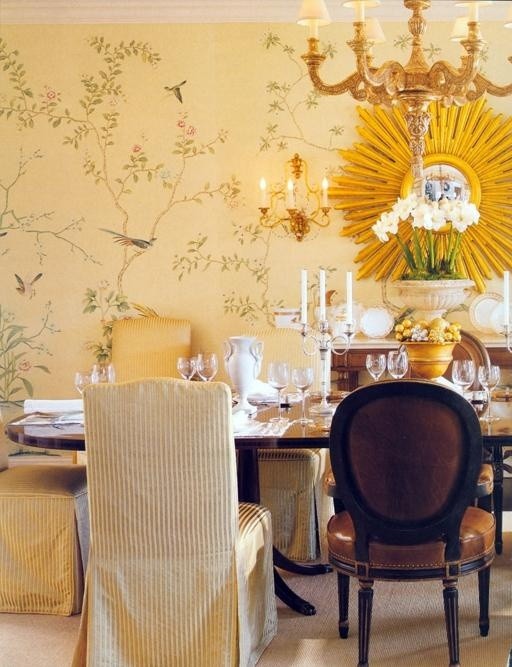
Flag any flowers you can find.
[394,317,462,344]
[372,194,481,278]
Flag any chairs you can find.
[327,378,495,667]
[107,319,192,389]
[0,414,89,616]
[255,448,327,561]
[72,374,278,665]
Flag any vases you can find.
[398,342,458,380]
[395,278,476,324]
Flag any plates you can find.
[360,307,394,339]
[469,292,505,335]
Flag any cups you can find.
[271,308,301,327]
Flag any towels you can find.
[436,375,463,398]
[232,408,247,434]
[251,381,277,397]
[23,397,87,413]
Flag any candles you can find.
[319,270,326,322]
[502,271,509,326]
[300,270,308,322]
[347,272,353,323]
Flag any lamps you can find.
[255,152,333,243]
[294,0,512,197]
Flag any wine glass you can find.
[452,360,476,392]
[292,368,313,425]
[366,354,387,381]
[177,358,196,381]
[268,362,290,423]
[92,363,116,386]
[197,353,218,381]
[388,351,409,379]
[478,365,501,421]
[74,371,100,395]
